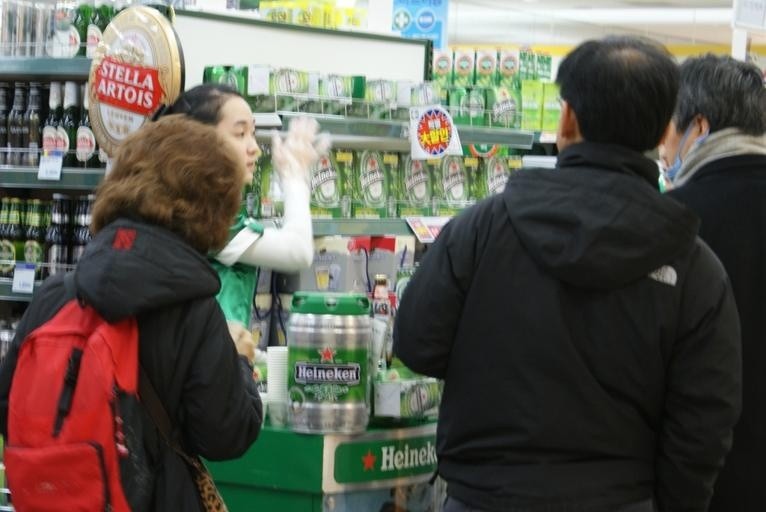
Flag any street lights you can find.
[192,460,227,512]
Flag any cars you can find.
[266,343,290,428]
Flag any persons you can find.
[660,55,766,512]
[394,34,743,512]
[0,113,262,512]
[152,83,332,338]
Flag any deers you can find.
[663,117,709,183]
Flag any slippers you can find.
[5,270,171,512]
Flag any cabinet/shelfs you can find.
[2,53,114,347]
[0,77,111,280]
[370,272,394,368]
[202,56,532,508]
